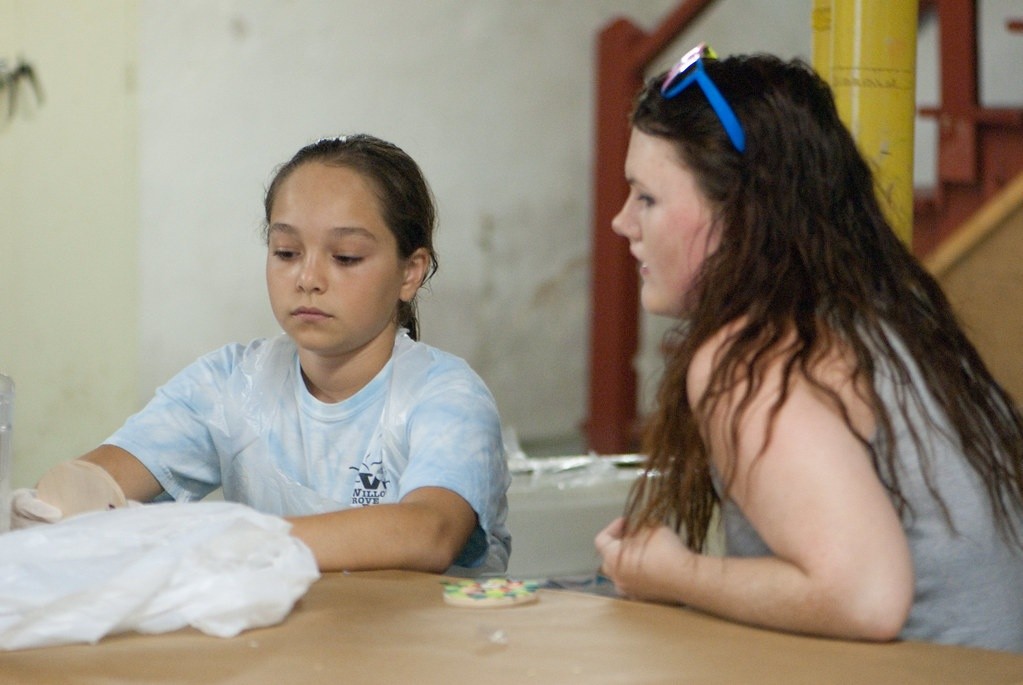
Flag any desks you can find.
[0,567,1023,685]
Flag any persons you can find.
[593,41,1023,657]
[7,134,514,581]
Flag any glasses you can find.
[664,42,749,153]
